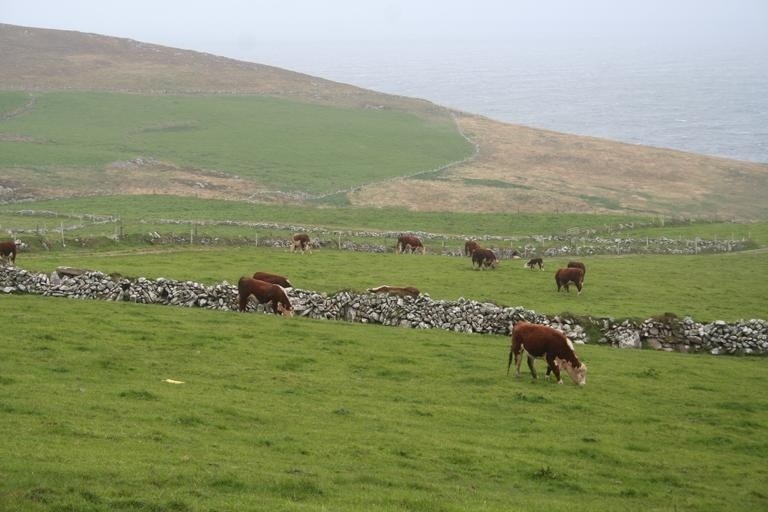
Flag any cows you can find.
[366,284,420,297]
[0,241,18,264]
[237,275,295,319]
[554,267,585,296]
[396,235,426,257]
[506,322,588,388]
[252,271,294,289]
[291,234,313,256]
[524,258,545,272]
[464,240,497,270]
[566,261,586,270]
